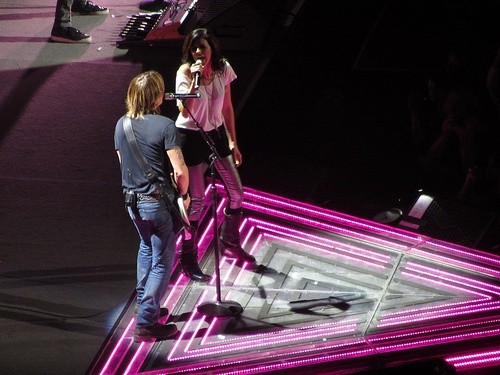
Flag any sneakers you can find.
[71,0,109,16]
[51,26,91,43]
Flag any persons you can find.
[409,39,500,215]
[176,28,255,280]
[115,71,191,342]
[51,0,109,42]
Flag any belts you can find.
[136,192,162,201]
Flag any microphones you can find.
[164,91,201,101]
[194,58,202,90]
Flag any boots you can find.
[180,219,208,282]
[218,208,255,262]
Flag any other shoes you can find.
[134,324,178,343]
[134,305,168,323]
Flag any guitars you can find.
[169,173,192,240]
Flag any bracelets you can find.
[182,193,188,200]
